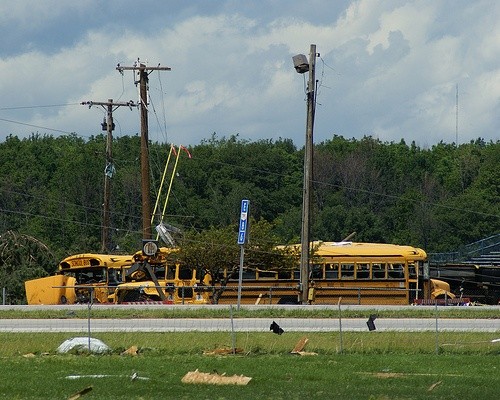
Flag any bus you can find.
[24,239,455,308]
